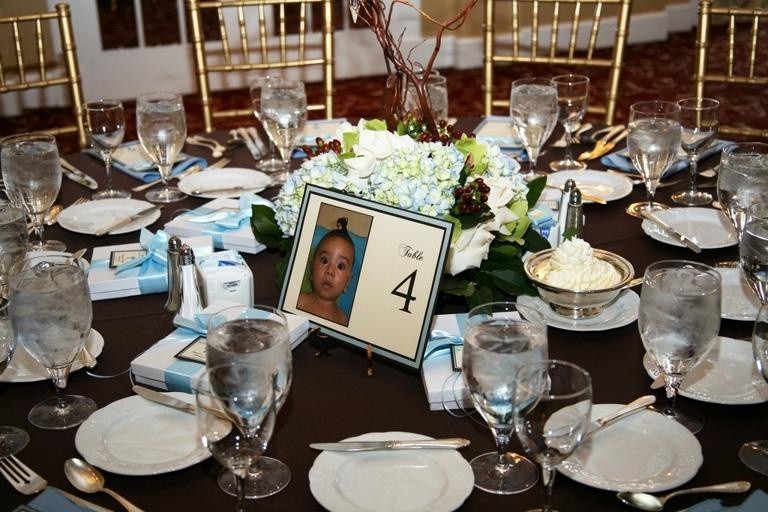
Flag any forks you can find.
[187,135,222,160]
[1,454,114,512]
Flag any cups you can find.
[250,79,280,169]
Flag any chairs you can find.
[691,3,767,140]
[482,2,631,124]
[2,3,93,151]
[189,0,335,136]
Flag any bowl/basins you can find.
[522,246,634,317]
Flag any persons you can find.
[296,217,354,328]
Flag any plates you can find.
[308,431,474,512]
[703,268,765,320]
[539,170,633,205]
[516,290,640,331]
[58,199,161,235]
[0,319,104,382]
[644,207,739,248]
[641,335,768,403]
[77,391,233,475]
[542,402,703,493]
[178,166,271,198]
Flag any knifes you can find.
[96,205,163,236]
[190,181,281,195]
[238,127,260,160]
[642,210,702,252]
[309,436,469,453]
[578,125,624,161]
[544,394,654,440]
[59,158,99,192]
[589,129,633,159]
[132,386,243,426]
[248,125,266,158]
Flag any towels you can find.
[82,140,207,183]
[601,126,739,179]
[13,486,95,511]
[677,489,768,511]
[471,115,528,162]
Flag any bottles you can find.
[176,244,203,322]
[163,238,182,313]
[564,188,584,243]
[558,179,576,244]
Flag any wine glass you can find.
[740,202,768,303]
[0,319,30,457]
[463,302,549,494]
[137,92,189,202]
[513,359,592,512]
[262,79,306,171]
[1,205,25,315]
[2,133,66,254]
[551,75,591,174]
[401,69,447,135]
[639,260,721,435]
[509,78,558,182]
[84,98,133,203]
[198,363,272,512]
[737,303,768,475]
[717,142,768,243]
[9,256,97,429]
[624,102,681,220]
[209,306,292,498]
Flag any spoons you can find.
[62,458,145,511]
[135,166,201,192]
[615,480,751,511]
[229,130,242,147]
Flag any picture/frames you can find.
[279,184,455,370]
